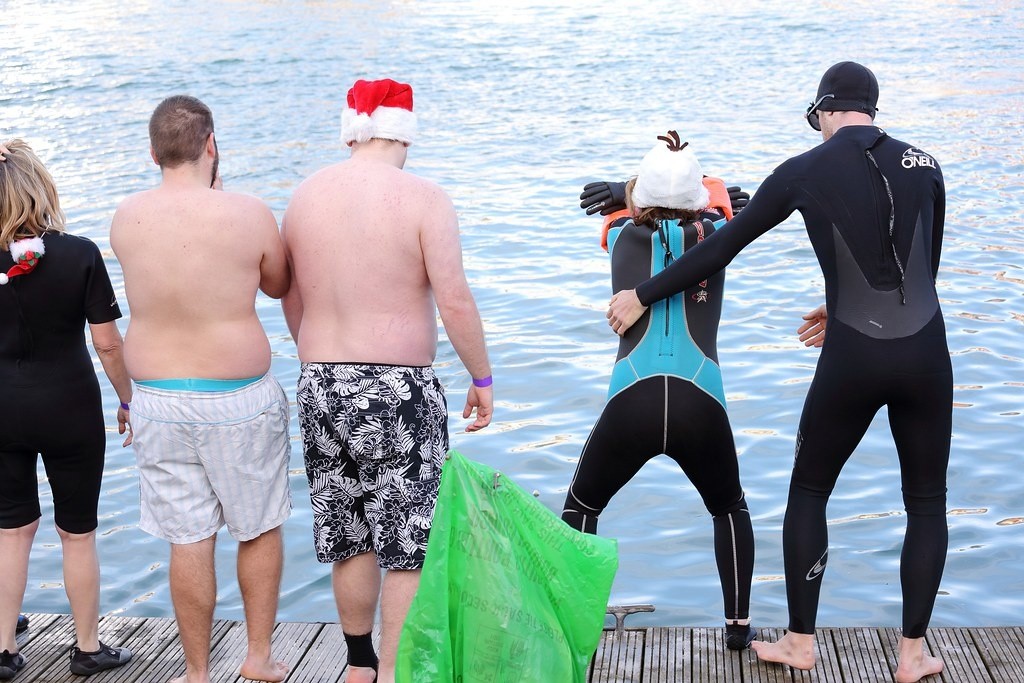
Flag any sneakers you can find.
[70,640,133,676]
[0,649,27,681]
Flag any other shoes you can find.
[14,614,29,638]
[725,621,757,650]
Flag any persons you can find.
[605,60,955,683]
[110,94,293,683]
[280,77,497,683]
[0,138,134,683]
[561,129,759,650]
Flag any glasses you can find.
[804,94,835,132]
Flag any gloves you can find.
[726,186,751,216]
[579,180,631,216]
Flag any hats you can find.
[341,77,416,144]
[630,129,710,211]
[815,61,880,114]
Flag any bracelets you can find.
[121,402,129,410]
[471,376,492,387]
